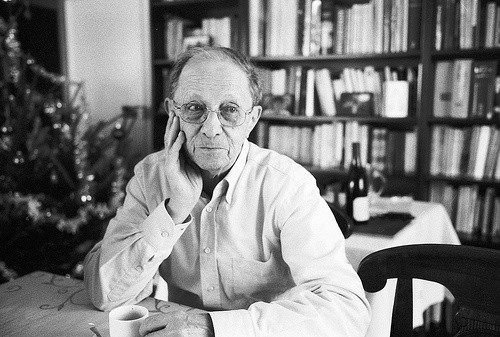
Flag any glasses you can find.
[172,100,253,127]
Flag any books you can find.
[258,120,499,181]
[158,0,499,59]
[251,58,500,117]
[427,183,499,245]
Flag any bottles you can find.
[345,140,371,225]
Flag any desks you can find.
[344,196,463,337]
[0,271,210,337]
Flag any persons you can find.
[84,46,371,336]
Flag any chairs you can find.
[357,245,500,337]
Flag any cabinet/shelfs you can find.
[147,0,500,247]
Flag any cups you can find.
[109,304,150,337]
